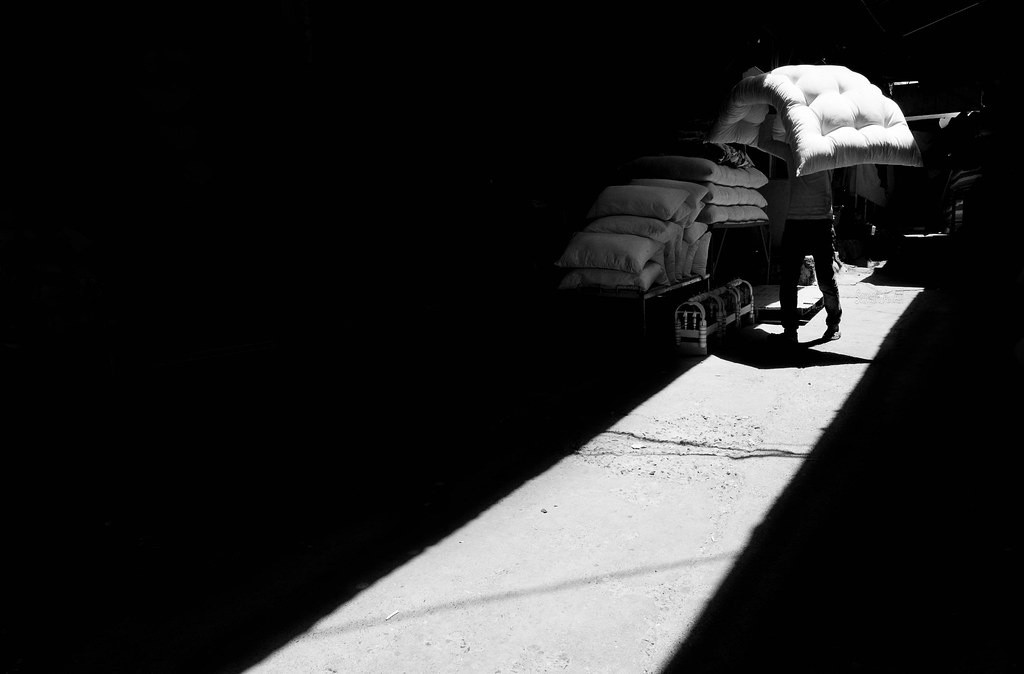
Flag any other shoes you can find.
[767,329,798,345]
[823,322,838,338]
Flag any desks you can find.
[701,221,773,286]
[597,274,712,342]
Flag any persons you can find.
[758,105,840,347]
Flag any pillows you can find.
[553,179,712,295]
